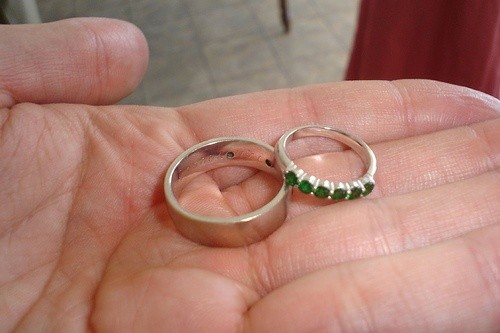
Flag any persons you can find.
[1,16,500,333]
